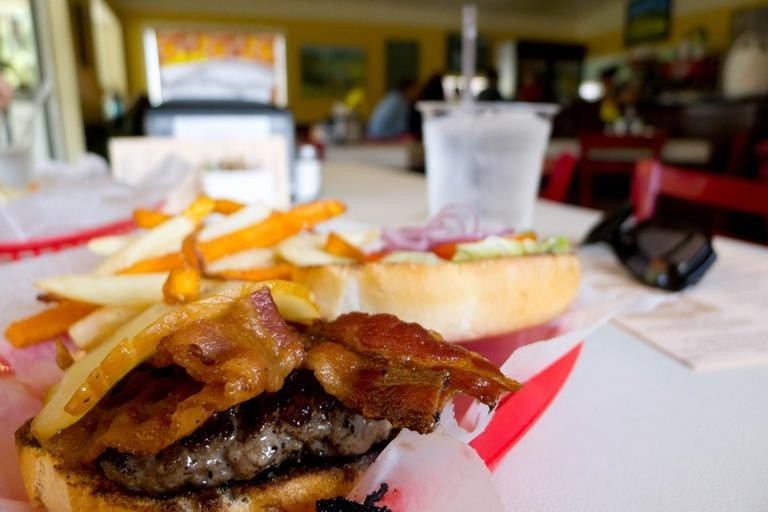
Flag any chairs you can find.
[538,127,767,260]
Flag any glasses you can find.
[580,200,718,293]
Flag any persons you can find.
[117,94,153,135]
[409,73,447,138]
[478,69,502,101]
[512,66,543,104]
[365,74,416,138]
[598,66,659,138]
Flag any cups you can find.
[410,96,562,243]
[0,97,37,191]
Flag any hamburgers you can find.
[8,198,581,512]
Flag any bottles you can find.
[294,142,324,211]
